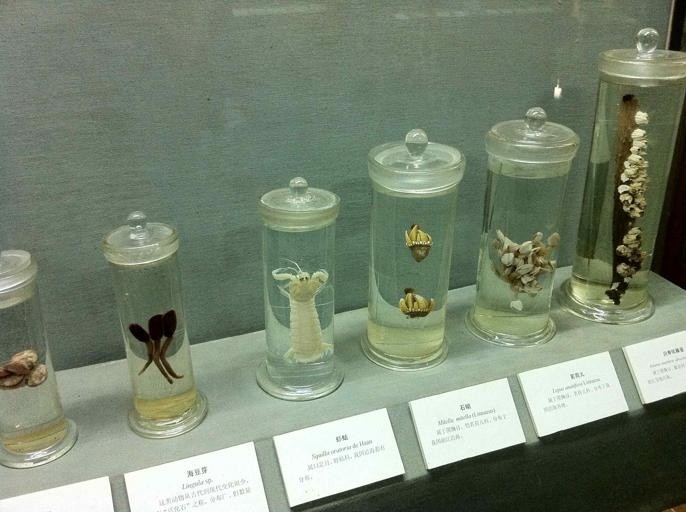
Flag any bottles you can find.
[553,27,686,326]
[463,106,580,349]
[360,127,466,373]
[103,210,209,439]
[0,249,78,468]
[256,175,346,402]
